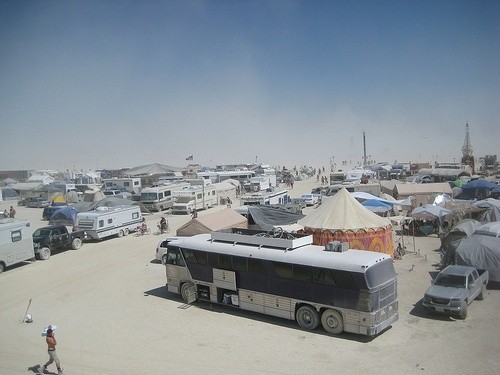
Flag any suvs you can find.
[103,190,122,197]
[42,207,60,220]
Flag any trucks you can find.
[0,218,35,273]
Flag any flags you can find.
[186,156,193,160]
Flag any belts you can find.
[48,349,55,352]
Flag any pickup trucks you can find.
[32,225,86,260]
[420,265,490,318]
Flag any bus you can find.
[161,227,399,337]
[330,172,345,184]
[139,170,276,215]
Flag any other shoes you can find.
[38,366,45,375]
[58,369,65,375]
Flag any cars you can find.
[25,198,50,208]
[291,198,307,209]
[17,197,35,206]
[300,194,319,206]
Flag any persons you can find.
[361,173,368,184]
[4,206,16,218]
[38,329,65,375]
[137,218,147,232]
[157,218,167,234]
[317,166,328,185]
[286,177,294,189]
[257,186,260,192]
[267,186,272,192]
[397,243,401,255]
[236,185,245,195]
[226,197,232,208]
[190,208,197,219]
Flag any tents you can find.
[2,189,19,200]
[0,177,17,188]
[297,185,395,261]
[382,179,453,205]
[33,184,64,200]
[48,206,79,226]
[176,208,248,236]
[409,205,456,237]
[460,179,496,200]
[65,189,106,205]
